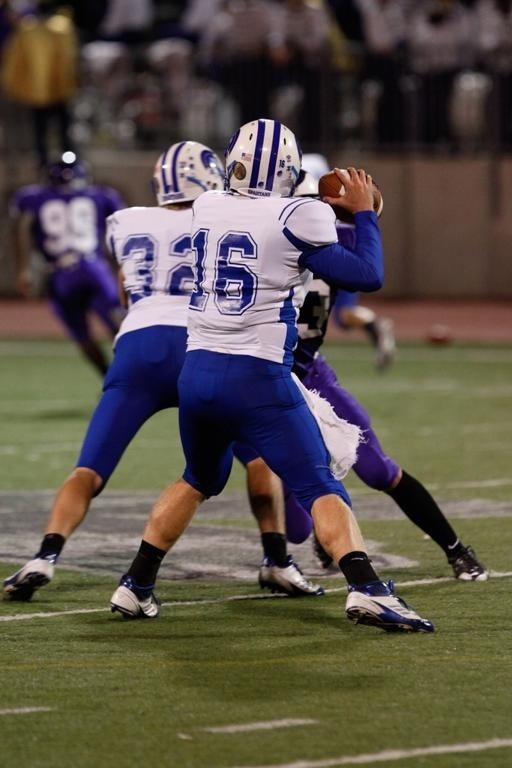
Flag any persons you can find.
[7,152,130,376]
[336,290,394,372]
[108,119,434,635]
[278,151,488,583]
[3,140,323,601]
[3,3,511,179]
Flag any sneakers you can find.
[450,546,487,581]
[260,555,325,597]
[347,579,435,630]
[109,575,161,618]
[3,558,55,601]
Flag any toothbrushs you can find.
[318,168,382,223]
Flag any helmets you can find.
[224,117,302,197]
[153,139,225,205]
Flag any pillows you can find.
[224,117,302,197]
[153,139,225,205]
